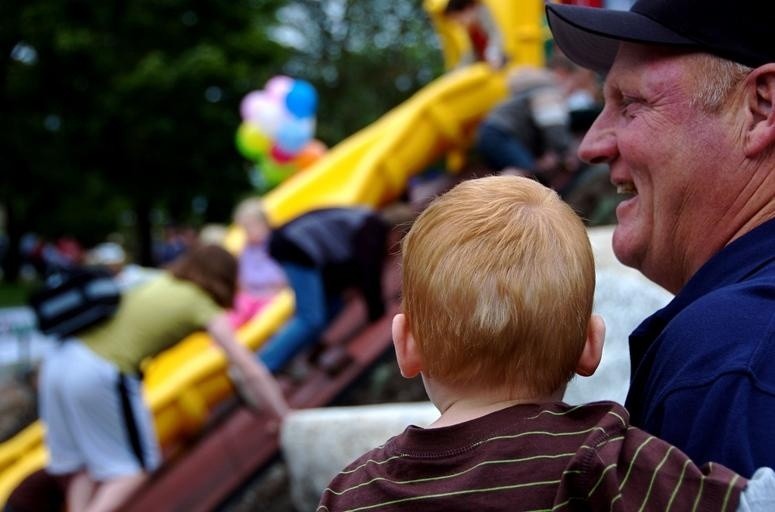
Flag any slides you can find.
[0,62,511,512]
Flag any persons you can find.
[223,199,292,329]
[441,0,508,71]
[476,50,595,184]
[33,244,296,510]
[227,200,420,410]
[309,173,775,511]
[540,0,775,473]
[80,241,144,289]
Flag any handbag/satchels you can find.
[29,263,119,335]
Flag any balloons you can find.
[237,75,330,195]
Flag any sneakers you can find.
[222,364,260,413]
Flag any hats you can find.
[546,1,774,74]
